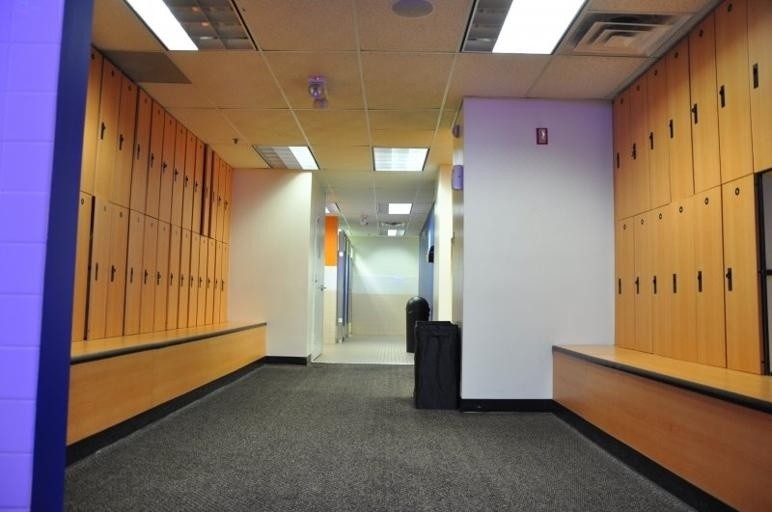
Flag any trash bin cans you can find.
[406,297,430,352]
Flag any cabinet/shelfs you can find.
[70,46,236,343]
[616,0,772,374]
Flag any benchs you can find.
[66,323,265,465]
[551,346,770,509]
